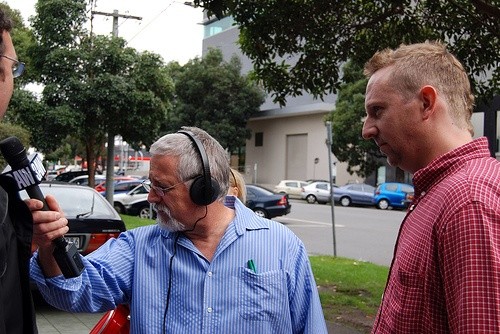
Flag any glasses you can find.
[140,175,201,197]
[0,54,26,78]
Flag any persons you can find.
[361,43,500,334]
[227,168,247,206]
[24,126,328,334]
[0,8,37,334]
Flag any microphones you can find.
[0,137,85,279]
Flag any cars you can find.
[19,183,126,310]
[245,184,292,220]
[373,182,414,210]
[273,180,309,200]
[333,184,377,206]
[301,182,338,204]
[46,164,157,220]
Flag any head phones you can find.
[176,130,220,205]
